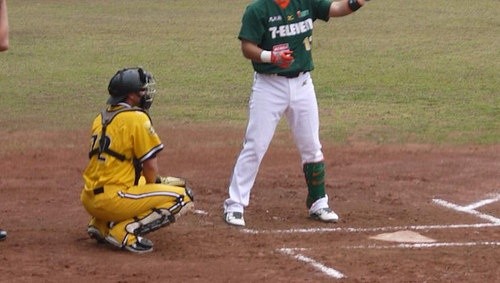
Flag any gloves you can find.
[271,49,296,68]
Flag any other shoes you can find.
[104,236,154,253]
[88,226,104,243]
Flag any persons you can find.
[81,67,194,253]
[0,0,9,240]
[223,0,373,226]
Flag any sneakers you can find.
[225,212,245,226]
[311,207,339,222]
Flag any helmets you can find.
[105,67,150,105]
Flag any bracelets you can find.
[348,0,361,11]
